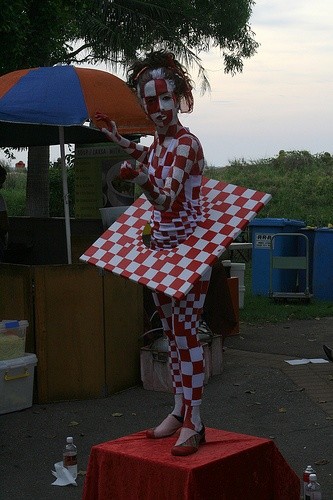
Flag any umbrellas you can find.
[0,65,156,264]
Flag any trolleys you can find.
[268,233,313,304]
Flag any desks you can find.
[92,423,282,500]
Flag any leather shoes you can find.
[146,413,183,439]
[170,423,206,456]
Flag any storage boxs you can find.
[221,260,246,309]
[0,319,38,414]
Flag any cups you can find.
[222,260,232,278]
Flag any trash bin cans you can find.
[249,218,306,300]
[308,223,333,302]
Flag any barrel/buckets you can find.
[231,262,245,309]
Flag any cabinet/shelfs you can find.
[221,277,239,336]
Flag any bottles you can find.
[303,466,321,500]
[63,437,78,480]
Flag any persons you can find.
[92,50,212,456]
